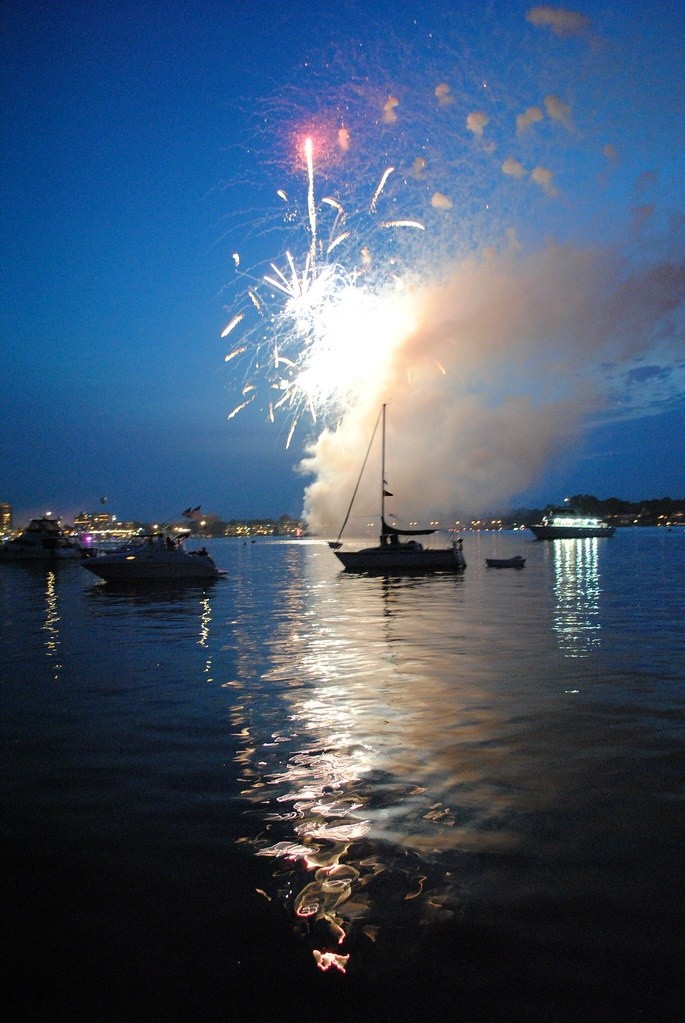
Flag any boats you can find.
[528,508,616,539]
[79,534,218,584]
[0,516,97,559]
[485,556,526,569]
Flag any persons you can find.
[166,536,186,552]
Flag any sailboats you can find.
[328,404,467,570]
